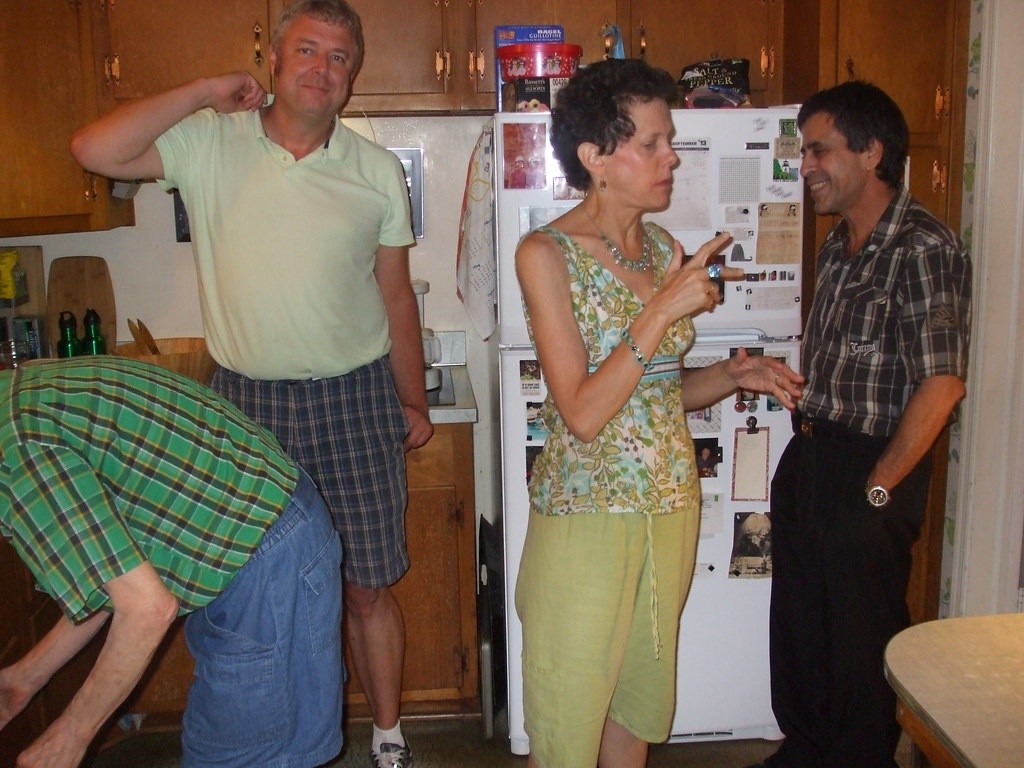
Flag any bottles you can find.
[57,306,106,358]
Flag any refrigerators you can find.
[491,104,804,755]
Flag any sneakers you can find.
[369,735,413,768]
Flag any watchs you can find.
[863,480,893,510]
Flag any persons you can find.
[766,80,966,768]
[0,356,347,768]
[695,448,715,471]
[73,0,437,768]
[512,56,806,768]
[742,527,770,556]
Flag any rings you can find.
[707,282,715,295]
[775,375,779,385]
[708,264,721,279]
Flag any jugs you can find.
[420,328,442,369]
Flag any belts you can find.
[790,412,891,450]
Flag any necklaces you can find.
[582,202,651,273]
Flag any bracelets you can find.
[620,329,654,371]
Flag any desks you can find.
[883,612,1023,767]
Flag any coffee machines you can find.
[410,281,443,391]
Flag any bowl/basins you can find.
[496,42,583,82]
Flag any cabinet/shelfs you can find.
[0,0,974,766]
